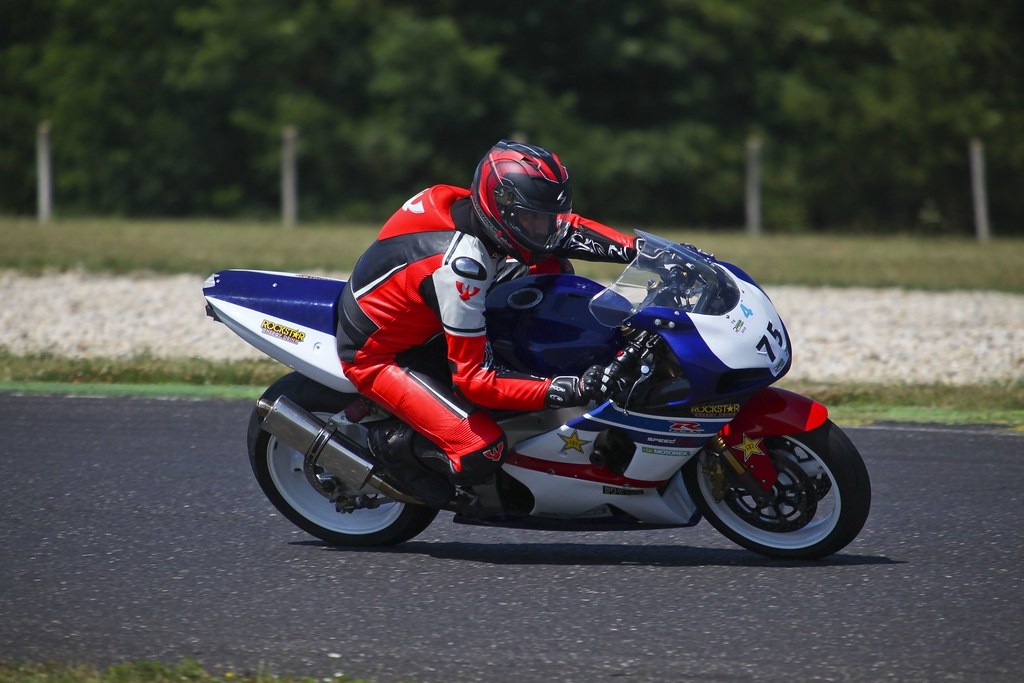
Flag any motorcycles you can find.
[197,229,877,563]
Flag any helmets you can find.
[471,139,572,265]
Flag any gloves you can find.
[634,236,699,288]
[544,363,626,409]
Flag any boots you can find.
[364,422,463,508]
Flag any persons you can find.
[337,139,698,504]
